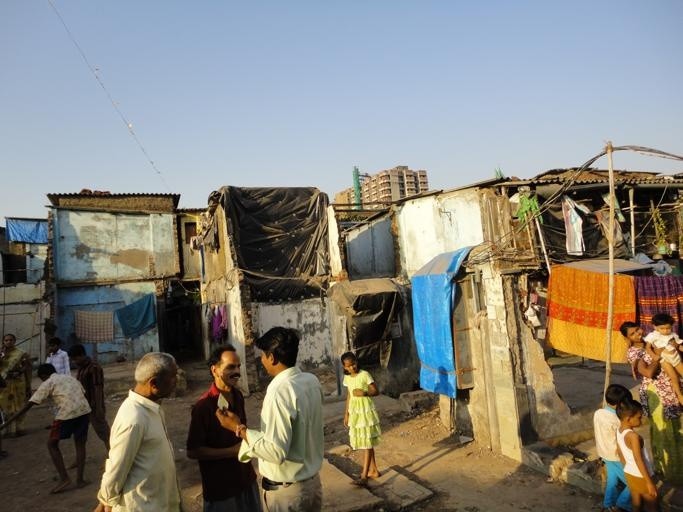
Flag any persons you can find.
[619,314,683,484]
[0,334,110,495]
[93,352,184,512]
[186,343,263,511]
[341,352,382,486]
[217,325,324,512]
[616,399,662,512]
[643,314,683,405]
[594,385,633,510]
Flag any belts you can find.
[262,476,293,491]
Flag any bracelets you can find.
[235,424,246,439]
